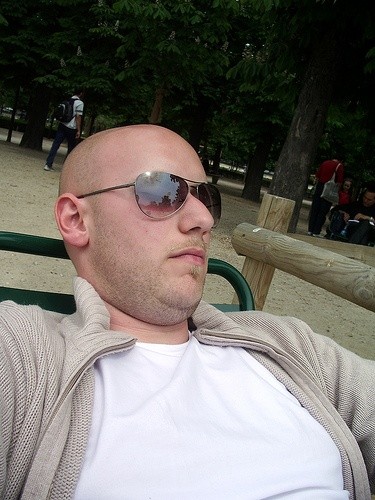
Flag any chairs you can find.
[0,231,256,334]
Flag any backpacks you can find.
[54,98,80,122]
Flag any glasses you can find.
[74,171,225,231]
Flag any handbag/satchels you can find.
[321,164,341,204]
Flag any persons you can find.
[306,153,344,238]
[324,177,375,247]
[1,125,375,500]
[43,86,85,172]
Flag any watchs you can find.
[370,217,373,222]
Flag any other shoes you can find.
[44,164,56,171]
[313,234,324,239]
[308,232,312,236]
